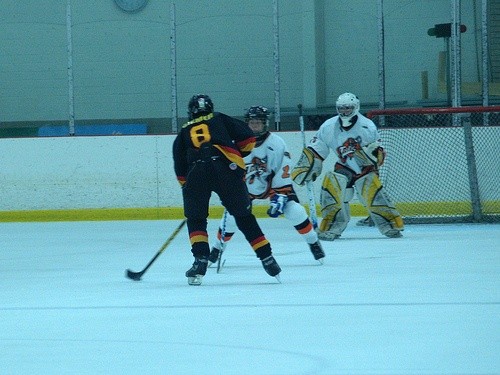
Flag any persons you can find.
[172,95,282,277]
[209,104,325,263]
[291,93,404,239]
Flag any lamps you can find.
[428,23,466,38]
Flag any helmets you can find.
[188,94,213,119]
[243,105,269,137]
[335,92,360,127]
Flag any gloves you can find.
[267,193,287,217]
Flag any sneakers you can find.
[186,257,209,284]
[310,240,326,264]
[262,255,282,283]
[208,248,223,263]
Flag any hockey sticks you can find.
[217,208,228,275]
[124,219,188,281]
[297,104,341,242]
